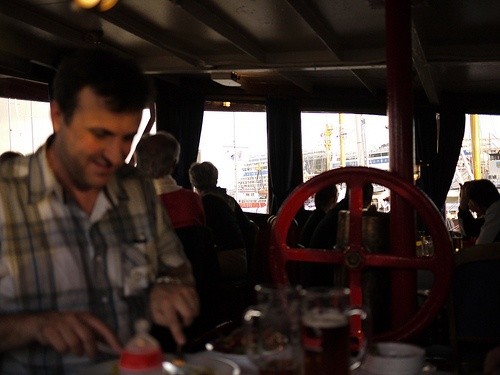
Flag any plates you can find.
[73,353,240,375]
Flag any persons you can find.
[134,132,206,230]
[300,180,373,288]
[189,162,259,282]
[483,346,500,375]
[1,46,199,374]
[460,179,500,246]
[294,184,337,250]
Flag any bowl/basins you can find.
[362,341,425,375]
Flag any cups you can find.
[452,229,463,254]
[298,287,367,374]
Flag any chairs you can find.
[173,225,222,311]
[444,239,500,375]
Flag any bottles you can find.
[116,319,164,374]
[416,235,434,257]
[243,285,303,375]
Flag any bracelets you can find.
[155,275,181,284]
